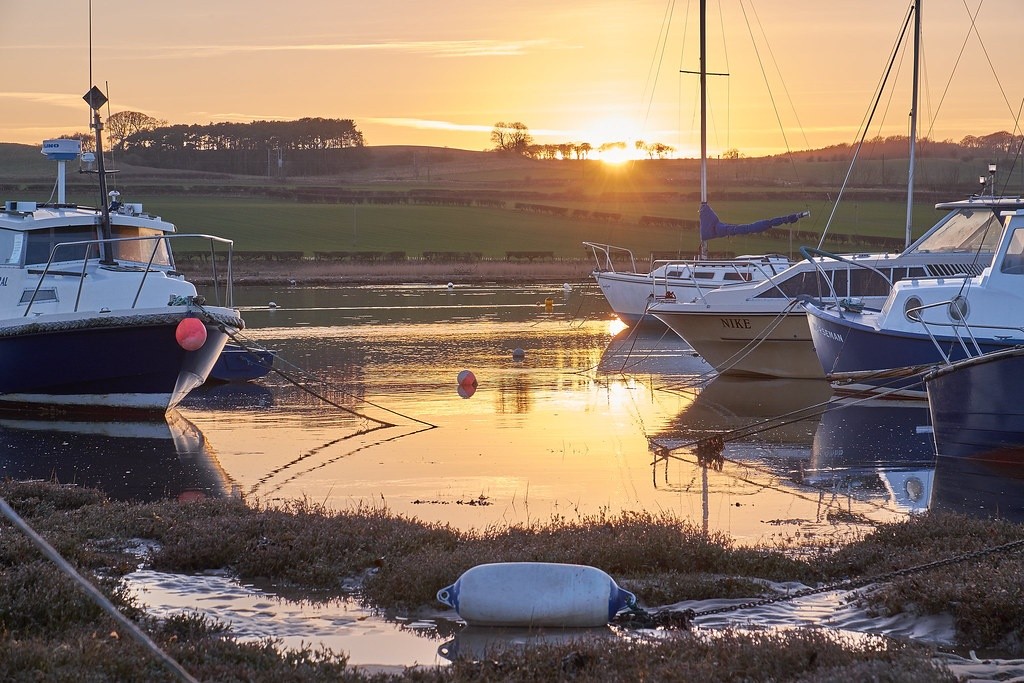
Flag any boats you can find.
[2,0,246,409]
[806,392,939,510]
[805,195,1024,398]
[176,380,276,413]
[203,342,273,381]
[921,341,1024,447]
[1,406,248,507]
[929,446,1024,527]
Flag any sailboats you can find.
[649,373,834,490]
[654,2,1021,382]
[596,330,721,380]
[588,1,794,326]
